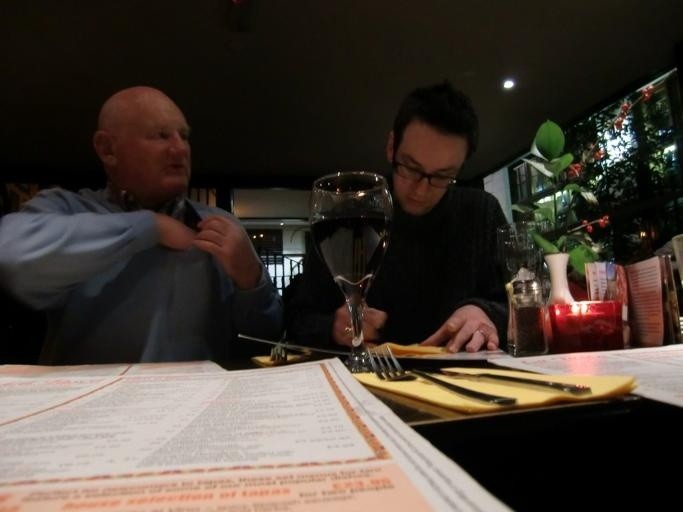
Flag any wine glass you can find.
[308,168,394,373]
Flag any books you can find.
[584,255,683,348]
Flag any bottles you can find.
[511,281,551,353]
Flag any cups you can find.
[546,300,625,354]
[499,219,551,278]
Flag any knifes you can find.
[409,365,593,397]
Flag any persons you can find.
[281,82,517,354]
[0,86,292,363]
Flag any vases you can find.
[542,251,578,338]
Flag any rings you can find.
[478,329,489,342]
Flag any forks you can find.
[364,342,516,409]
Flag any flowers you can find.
[525,80,667,275]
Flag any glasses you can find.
[392,139,458,187]
